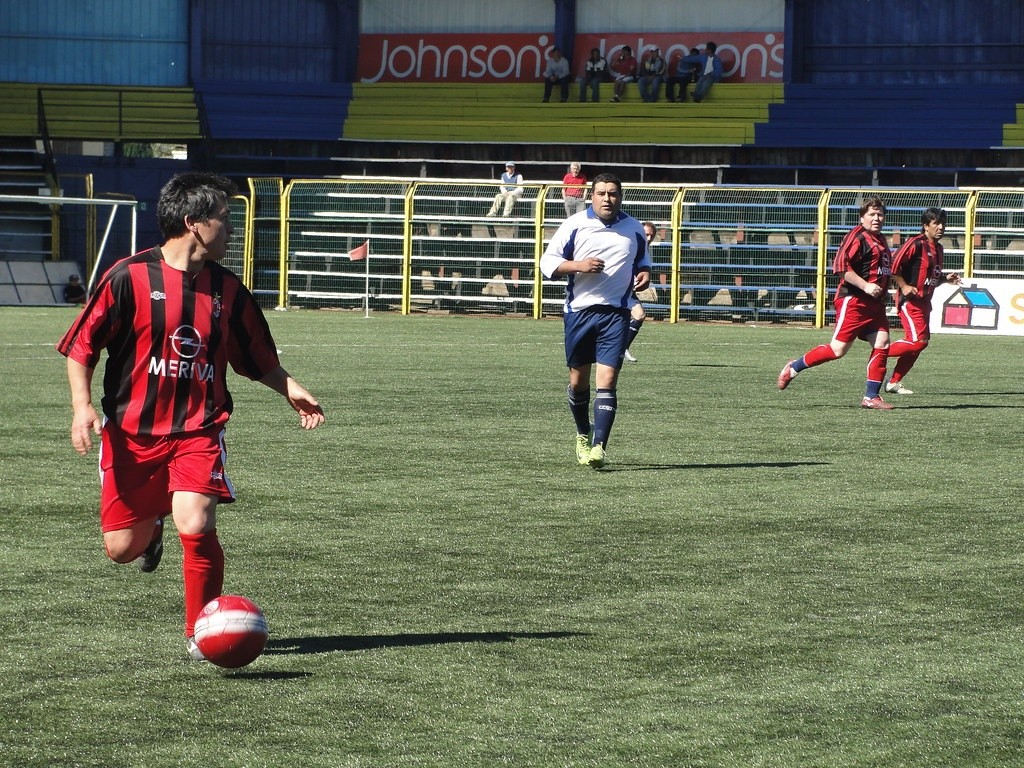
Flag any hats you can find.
[650,45,659,52]
[506,161,515,167]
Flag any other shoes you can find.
[675,97,681,102]
[503,214,510,218]
[543,98,548,101]
[694,97,699,102]
[486,213,497,217]
[610,95,620,102]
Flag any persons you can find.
[665,48,702,103]
[53,171,325,662]
[858,207,963,396]
[677,42,723,103]
[539,173,651,469]
[542,47,572,102]
[63,274,86,305]
[578,47,609,102]
[610,46,638,102]
[639,46,666,103]
[486,162,524,218]
[779,196,893,409]
[561,162,588,218]
[624,221,656,361]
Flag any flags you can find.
[349,241,367,260]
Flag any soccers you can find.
[193,594,269,669]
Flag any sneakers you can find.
[885,378,913,395]
[186,638,208,662]
[586,444,606,468]
[575,421,596,464]
[862,397,895,411]
[625,350,638,364]
[778,358,796,389]
[139,517,165,573]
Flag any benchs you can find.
[0,72,1023,329]
